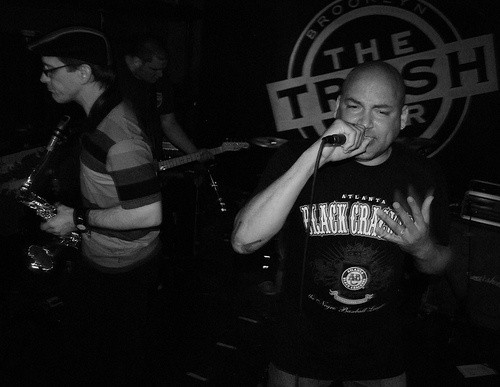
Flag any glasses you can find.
[39,62,76,76]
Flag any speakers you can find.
[446,218,500,330]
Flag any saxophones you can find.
[12,112,81,272]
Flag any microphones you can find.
[321,133,346,146]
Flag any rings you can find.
[400,228,405,237]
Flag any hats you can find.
[26,26,111,67]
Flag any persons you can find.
[104,34,214,162]
[230,60,469,387]
[28,26,163,387]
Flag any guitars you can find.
[158,141,250,170]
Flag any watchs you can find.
[74,206,90,234]
